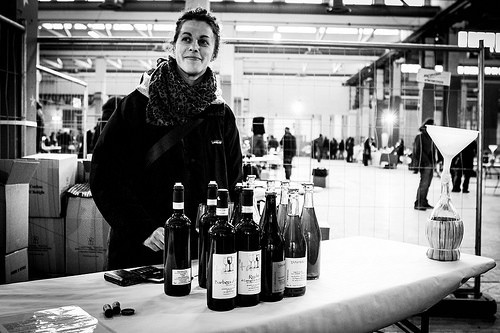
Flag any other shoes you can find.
[414,200,426,210]
[421,198,434,209]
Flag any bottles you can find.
[425,179,464,261]
[163,175,321,312]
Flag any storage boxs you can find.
[0,153,111,284]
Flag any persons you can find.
[41,117,101,158]
[312,133,375,167]
[439,143,476,193]
[91,97,123,148]
[34,102,45,153]
[280,128,296,179]
[89,7,243,272]
[411,118,437,210]
[396,139,404,163]
[268,135,278,169]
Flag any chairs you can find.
[378,153,398,169]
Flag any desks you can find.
[0,237,496,333]
